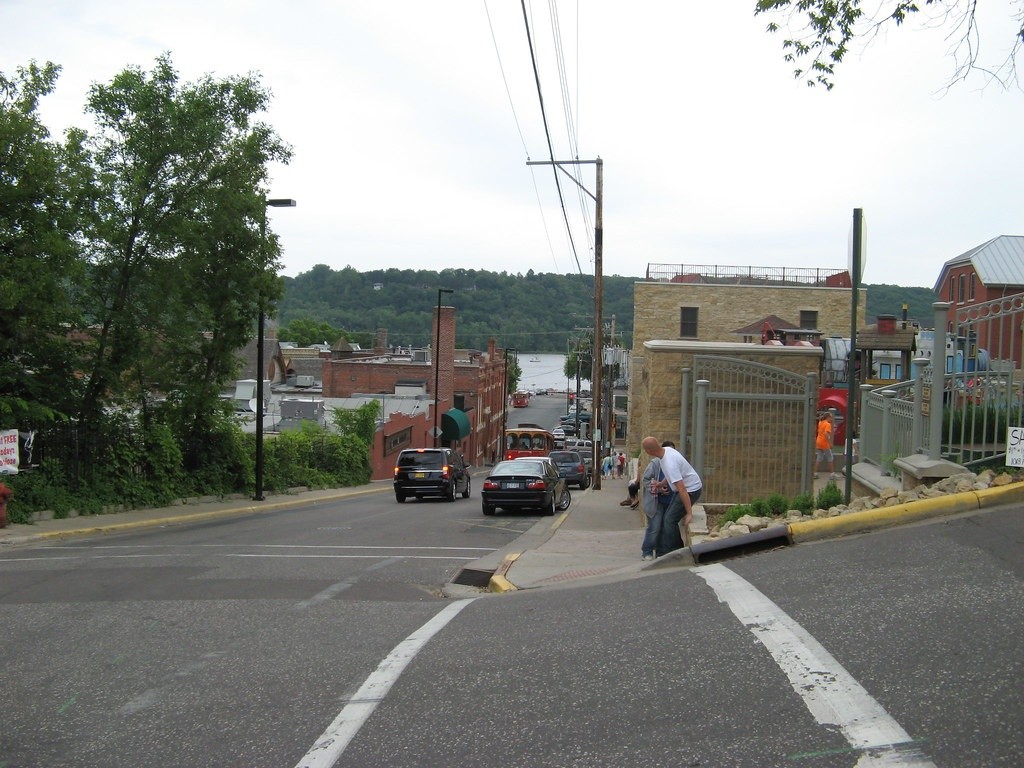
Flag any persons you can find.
[842,432,858,476]
[641,441,675,561]
[642,437,702,558]
[611,452,619,479]
[813,412,836,480]
[600,456,604,475]
[602,452,611,480]
[617,453,624,479]
[620,455,641,510]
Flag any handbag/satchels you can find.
[617,459,621,466]
[607,464,613,470]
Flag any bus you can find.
[512,391,529,408]
[503,423,555,463]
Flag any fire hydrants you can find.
[0,483,12,528]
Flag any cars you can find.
[481,458,571,515]
[549,450,591,490]
[551,402,593,474]
[514,456,567,478]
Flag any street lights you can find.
[248,198,297,501]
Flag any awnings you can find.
[441,409,470,439]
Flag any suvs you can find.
[393,447,472,502]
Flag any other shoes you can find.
[813,473,820,480]
[830,472,841,480]
[642,551,654,563]
[630,499,640,510]
[621,499,632,506]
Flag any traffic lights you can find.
[569,394,574,400]
